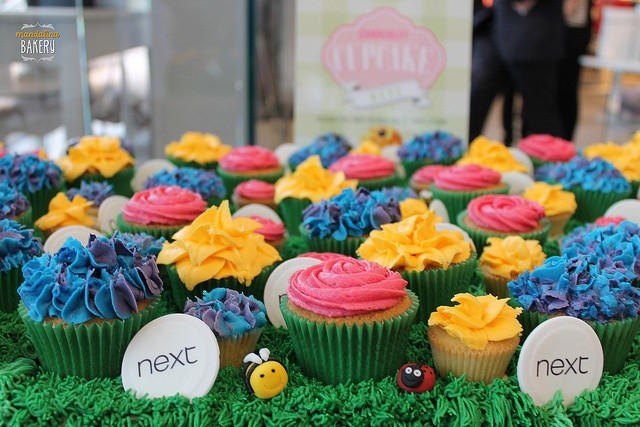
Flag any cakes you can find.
[397,128,463,178]
[0,219,44,313]
[0,148,64,213]
[583,129,640,199]
[356,197,478,324]
[231,179,275,212]
[278,252,419,385]
[0,184,34,228]
[301,186,400,263]
[52,133,136,196]
[181,288,267,369]
[140,166,228,208]
[64,179,116,208]
[375,184,418,202]
[522,175,576,234]
[506,253,640,374]
[535,147,634,221]
[427,291,524,386]
[544,211,640,283]
[286,133,352,173]
[216,145,284,206]
[116,185,209,242]
[430,163,510,226]
[230,203,285,252]
[163,132,232,172]
[516,133,577,166]
[271,154,360,235]
[474,236,548,298]
[328,151,400,191]
[457,192,552,255]
[407,164,448,202]
[155,200,282,313]
[452,133,532,186]
[34,192,98,231]
[16,230,165,380]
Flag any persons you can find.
[497,1,592,143]
[469,0,512,143]
